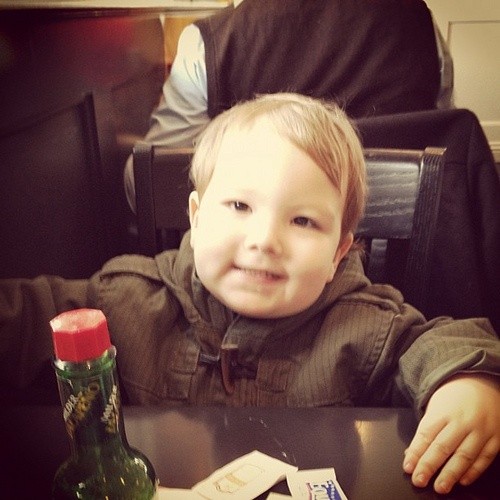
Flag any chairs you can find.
[132,107,500,332]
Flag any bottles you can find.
[49,309,160,500]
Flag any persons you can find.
[122,0,453,218]
[1,87,500,494]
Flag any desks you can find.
[0,407,500,500]
